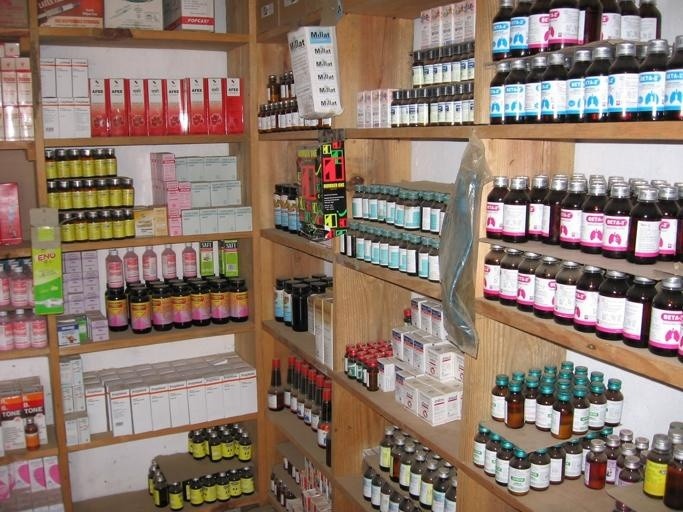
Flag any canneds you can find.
[273,272,332,332]
[104,274,247,334]
[272,184,299,234]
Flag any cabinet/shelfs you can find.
[0,1,274,512]
[250,0,682,512]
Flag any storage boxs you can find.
[256,1,280,33]
[274,0,326,26]
[164,1,216,32]
[1,1,29,27]
[104,0,165,30]
[38,0,105,29]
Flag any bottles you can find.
[268,455,300,507]
[24,416,41,452]
[272,271,332,331]
[613,422,683,512]
[105,251,125,331]
[272,182,299,235]
[188,422,253,462]
[339,222,441,284]
[490,361,623,440]
[143,246,157,281]
[48,176,136,210]
[342,340,392,391]
[363,425,456,511]
[283,355,330,467]
[490,1,660,61]
[257,73,331,134]
[267,358,283,410]
[124,247,138,283]
[148,458,254,511]
[483,244,683,366]
[126,275,249,335]
[489,35,682,125]
[182,242,196,277]
[161,245,175,278]
[486,174,682,265]
[58,207,136,244]
[472,424,612,496]
[390,41,474,127]
[44,149,116,181]
[351,185,450,237]
[0,259,48,353]
